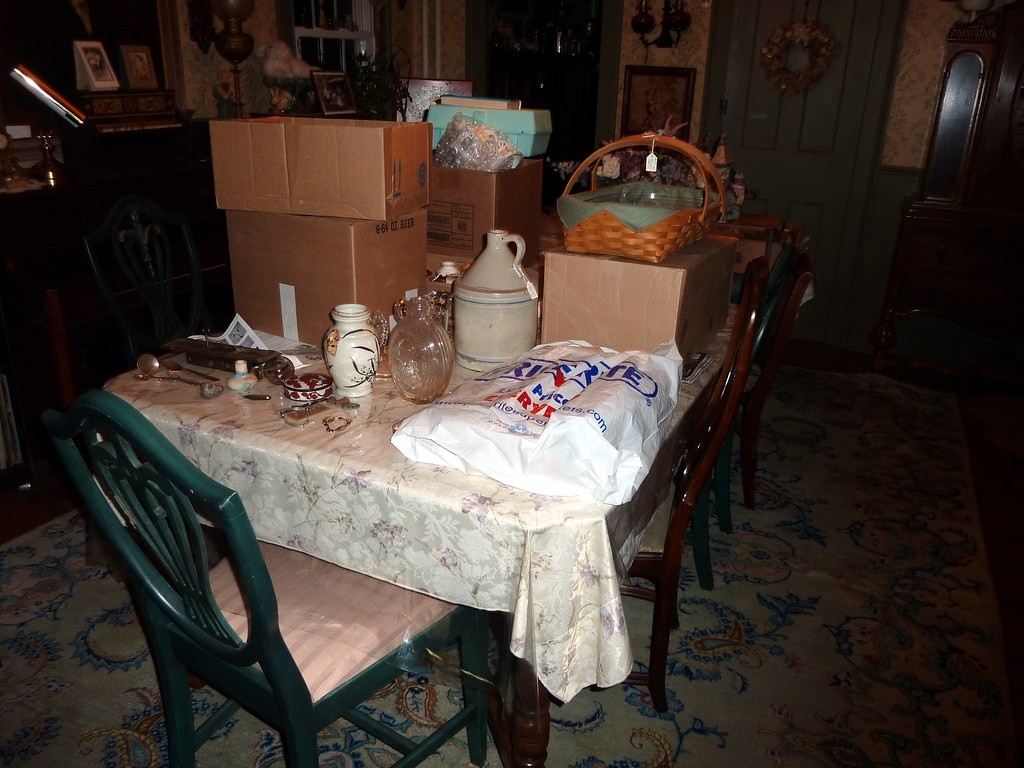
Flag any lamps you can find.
[632,0,692,48]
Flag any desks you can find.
[102,236,784,768]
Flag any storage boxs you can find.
[426,104,552,258]
[226,208,427,347]
[557,135,726,263]
[541,234,739,360]
[209,119,432,221]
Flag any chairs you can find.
[84,193,210,365]
[619,215,814,712]
[31,392,490,768]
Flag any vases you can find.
[311,69,357,117]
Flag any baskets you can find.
[560,134,727,264]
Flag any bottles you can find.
[386,297,456,407]
[439,262,459,277]
[322,302,381,398]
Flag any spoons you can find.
[162,357,221,382]
[166,369,225,400]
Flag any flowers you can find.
[762,17,834,92]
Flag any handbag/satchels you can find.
[390,336,683,505]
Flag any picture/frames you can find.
[621,65,696,143]
[122,46,158,89]
[76,41,121,86]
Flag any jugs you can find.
[450,228,540,373]
